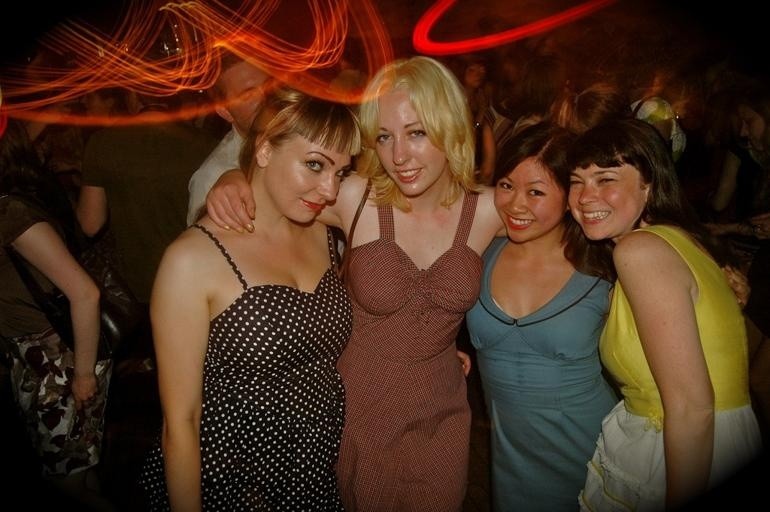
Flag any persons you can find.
[2,34,757,488]
[466,122,615,512]
[135,87,470,510]
[205,57,750,510]
[559,116,762,509]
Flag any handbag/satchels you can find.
[52,256,146,364]
